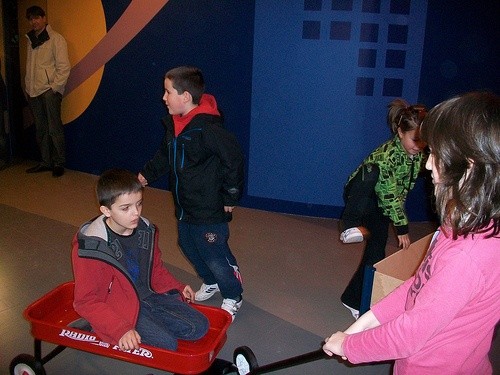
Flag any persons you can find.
[137,66,244,325]
[322,91,500,375]
[67,168,209,352]
[339,99,428,320]
[24,6,71,177]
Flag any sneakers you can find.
[220,294,243,323]
[195,283,220,301]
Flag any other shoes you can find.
[340,227,363,243]
[51,167,65,177]
[67,318,93,332]
[25,164,49,173]
[342,303,359,320]
